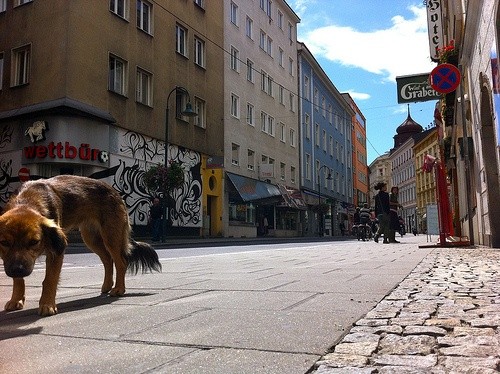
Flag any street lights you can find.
[161,86,198,241]
[317,164,334,236]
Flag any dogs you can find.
[0,175,163,318]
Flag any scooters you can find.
[399,224,404,238]
[352,223,372,241]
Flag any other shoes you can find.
[383,240,389,244]
[389,239,400,243]
[374,235,379,243]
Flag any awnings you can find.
[228,172,307,211]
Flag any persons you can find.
[373,182,389,242]
[389,186,405,242]
[352,204,379,240]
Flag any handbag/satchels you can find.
[383,215,391,224]
